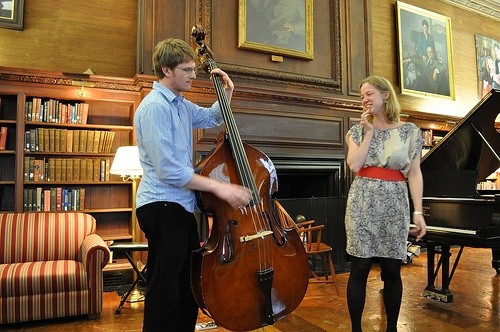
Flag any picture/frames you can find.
[395,0,456,102]
[237,0,314,62]
[0,0,26,32]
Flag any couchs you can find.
[0,212,110,325]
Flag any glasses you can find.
[175,65,198,74]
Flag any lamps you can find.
[109,145,147,303]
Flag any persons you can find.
[135,38,253,332]
[342,75,426,332]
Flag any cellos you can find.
[190,24,310,332]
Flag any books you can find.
[421,129,444,157]
[24,186,86,211]
[24,128,115,153]
[25,97,90,125]
[24,157,110,182]
[0,126,7,150]
[477,181,496,190]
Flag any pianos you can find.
[406,89,500,303]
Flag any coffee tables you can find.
[108,241,149,315]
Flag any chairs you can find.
[295,219,340,297]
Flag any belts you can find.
[354,165,404,183]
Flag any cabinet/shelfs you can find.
[0,65,141,293]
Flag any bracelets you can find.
[414,211,422,215]
